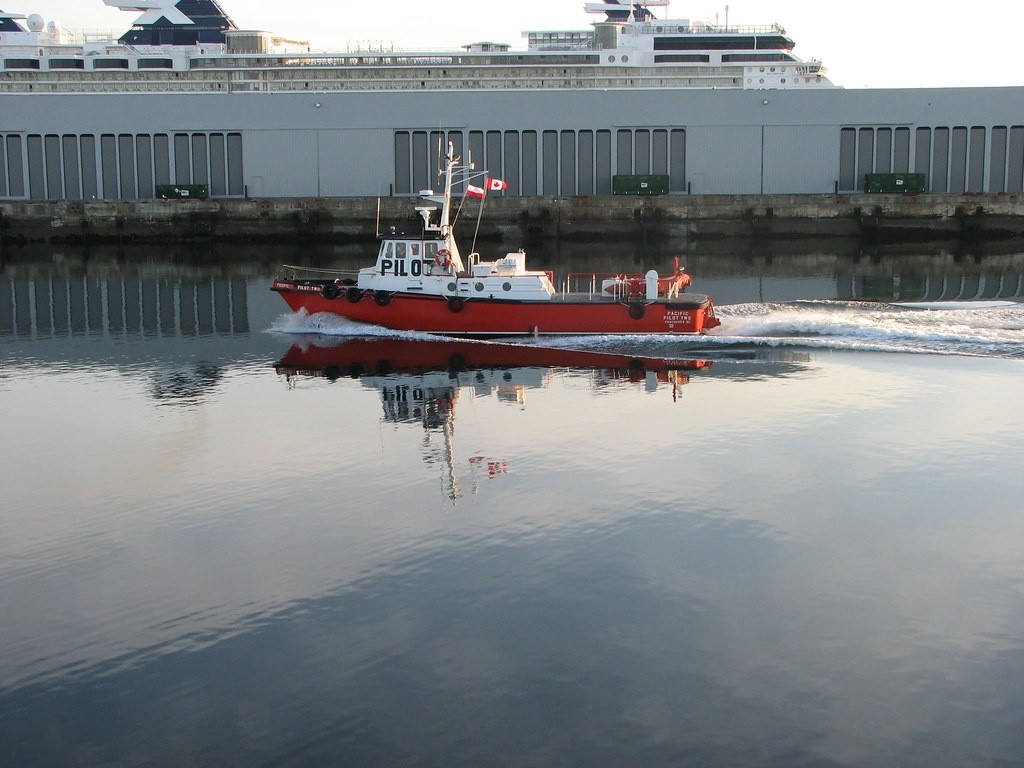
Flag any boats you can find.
[272,334,713,506]
[1,0,843,89]
[269,119,723,336]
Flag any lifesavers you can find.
[629,302,645,320]
[323,284,338,299]
[435,249,452,267]
[374,291,390,307]
[346,287,363,303]
[447,296,464,313]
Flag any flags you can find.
[487,179,508,190]
[468,457,484,464]
[466,184,488,199]
[488,461,508,477]
